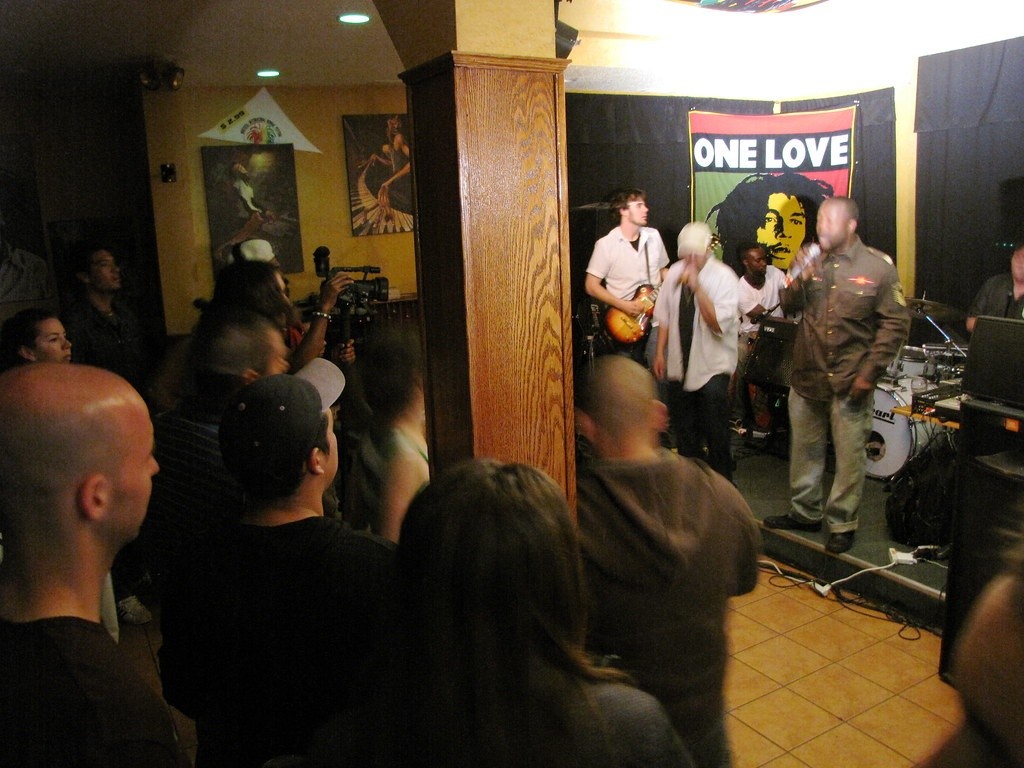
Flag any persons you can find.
[583,188,671,451]
[966,244,1024,332]
[356,113,413,215]
[762,195,909,554]
[0,246,758,768]
[651,221,739,487]
[728,241,789,471]
[902,497,1024,768]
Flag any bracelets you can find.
[313,311,331,319]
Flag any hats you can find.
[215,356,347,487]
[676,221,713,257]
[228,240,279,270]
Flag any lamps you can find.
[139,61,185,91]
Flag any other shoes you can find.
[764,514,823,533]
[826,530,855,552]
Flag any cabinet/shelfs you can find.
[939,397,1023,688]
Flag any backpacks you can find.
[885,429,961,548]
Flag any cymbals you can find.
[904,296,966,321]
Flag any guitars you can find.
[600,232,723,348]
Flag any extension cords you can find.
[809,581,828,595]
[889,548,897,565]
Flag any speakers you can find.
[574,202,623,285]
[744,319,798,395]
[937,313,1024,691]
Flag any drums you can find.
[885,345,929,377]
[866,375,940,481]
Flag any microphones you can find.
[335,265,381,274]
[750,304,780,324]
[781,244,821,288]
